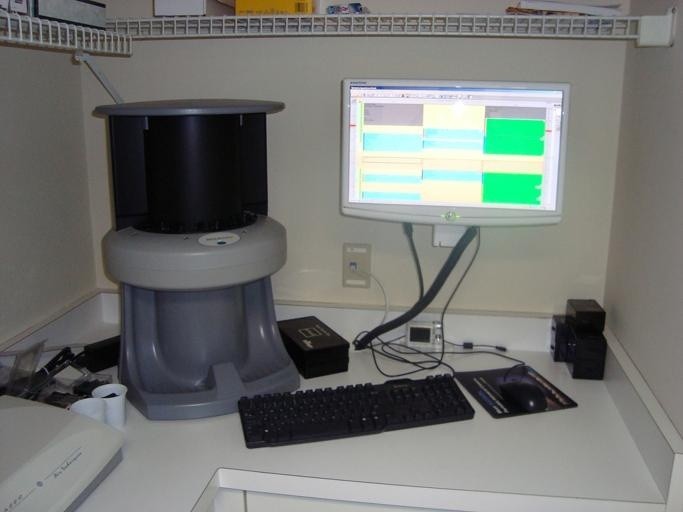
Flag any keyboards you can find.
[237,372,475,449]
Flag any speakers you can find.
[549,298,608,381]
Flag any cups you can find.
[89,382,129,427]
[69,397,107,425]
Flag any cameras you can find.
[406,320,445,348]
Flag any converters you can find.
[83,334,121,374]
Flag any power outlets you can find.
[342,242,372,287]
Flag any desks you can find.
[62,324,683,512]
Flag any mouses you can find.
[500,382,548,414]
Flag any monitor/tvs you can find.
[341,78,571,226]
